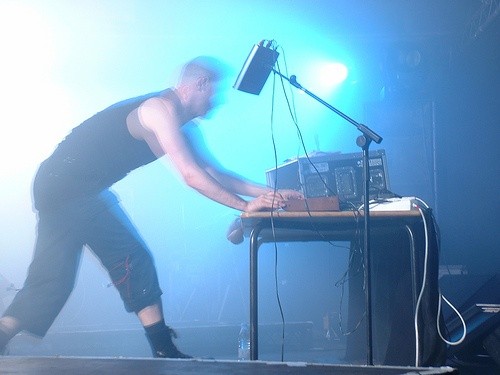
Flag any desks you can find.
[241,211,424,366]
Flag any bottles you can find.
[237,322,250,362]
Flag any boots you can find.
[149,327,190,358]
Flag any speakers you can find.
[441,271,500,374]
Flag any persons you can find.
[0,58,304,358]
